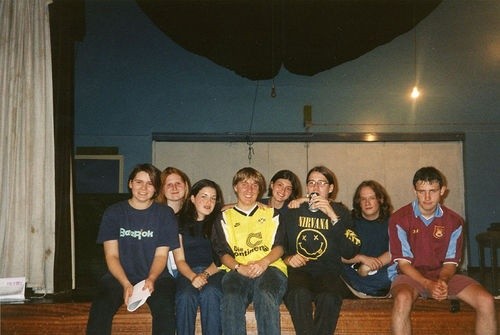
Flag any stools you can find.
[475,228,498,287]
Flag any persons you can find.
[86,163,181,335]
[211,167,288,335]
[172,179,225,335]
[281,165,363,335]
[262,170,310,225]
[338,180,394,299]
[388,166,497,335]
[154,166,265,217]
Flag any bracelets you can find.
[204,271,210,279]
[331,216,342,226]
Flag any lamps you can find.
[266,0,277,96]
[404,1,424,102]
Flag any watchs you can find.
[235,263,243,272]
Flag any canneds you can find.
[308,192,320,212]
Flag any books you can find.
[127,279,151,312]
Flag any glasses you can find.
[307,180,329,186]
